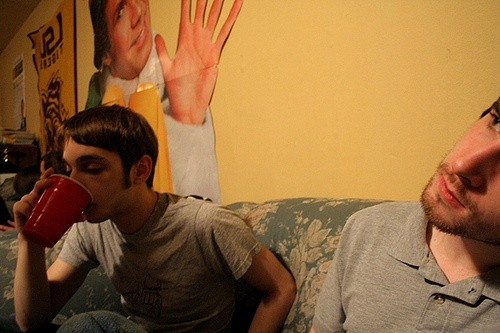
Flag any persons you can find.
[13,105,297,333]
[84,0,245,205]
[309,98,500,333]
[0,152,65,232]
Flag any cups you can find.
[22,173,92,248]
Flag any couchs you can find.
[0,197,394,333]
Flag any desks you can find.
[0,144,39,172]
